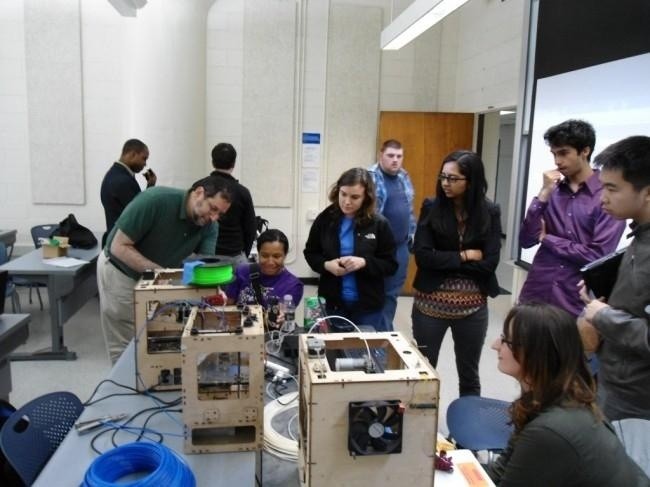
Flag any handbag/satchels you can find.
[52,213,97,248]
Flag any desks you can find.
[0,247,102,360]
[0,313,31,403]
[30,337,439,487]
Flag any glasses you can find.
[438,172,467,182]
[500,332,526,347]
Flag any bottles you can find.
[282,295,296,332]
[267,295,281,323]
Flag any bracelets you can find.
[464,250,468,261]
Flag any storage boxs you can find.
[42,236,71,258]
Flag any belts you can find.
[107,257,127,274]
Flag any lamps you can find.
[380,0,468,51]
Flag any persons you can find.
[304,168,399,331]
[101,139,157,233]
[96,177,233,366]
[192,143,256,264]
[480,302,650,485]
[368,139,417,329]
[518,119,628,315]
[578,134,650,472]
[228,229,304,310]
[414,150,502,396]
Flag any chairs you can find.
[447,396,517,470]
[0,228,19,313]
[30,224,59,310]
[0,392,85,487]
